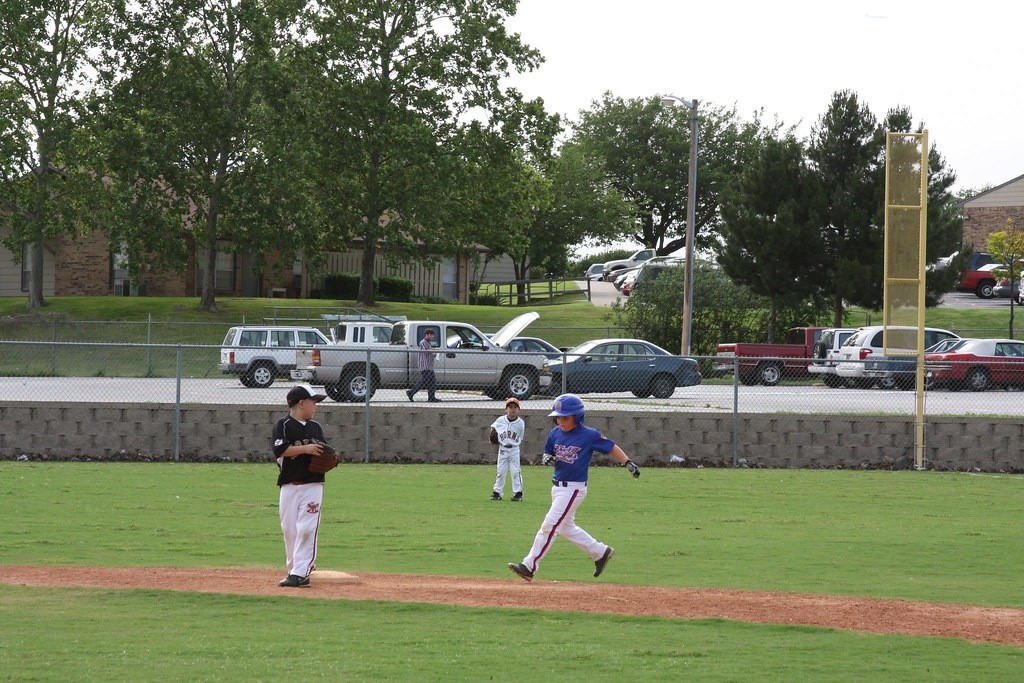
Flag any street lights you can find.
[663,94,700,354]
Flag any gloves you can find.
[540,455,556,467]
[625,459,640,479]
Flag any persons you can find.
[271,385,328,587]
[406,328,441,402]
[508,393,641,582]
[489,397,524,501]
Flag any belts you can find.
[289,481,305,485]
[551,479,587,487]
[500,444,512,448]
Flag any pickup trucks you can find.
[712,327,831,387]
[863,338,975,390]
[289,310,553,401]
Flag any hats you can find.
[286,385,327,408]
[506,398,519,408]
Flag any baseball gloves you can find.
[308,437,338,474]
[489,429,498,444]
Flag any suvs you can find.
[807,327,862,388]
[835,325,964,390]
[217,326,335,388]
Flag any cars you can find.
[581,247,719,295]
[918,338,1024,393]
[539,338,703,398]
[446,332,564,399]
[925,250,1024,307]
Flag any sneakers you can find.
[593,547,615,577]
[489,492,502,500]
[277,575,311,587]
[511,492,523,501]
[508,563,534,582]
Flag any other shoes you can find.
[428,397,441,402]
[406,391,414,402]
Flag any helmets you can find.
[547,394,586,427]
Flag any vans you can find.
[330,322,395,345]
